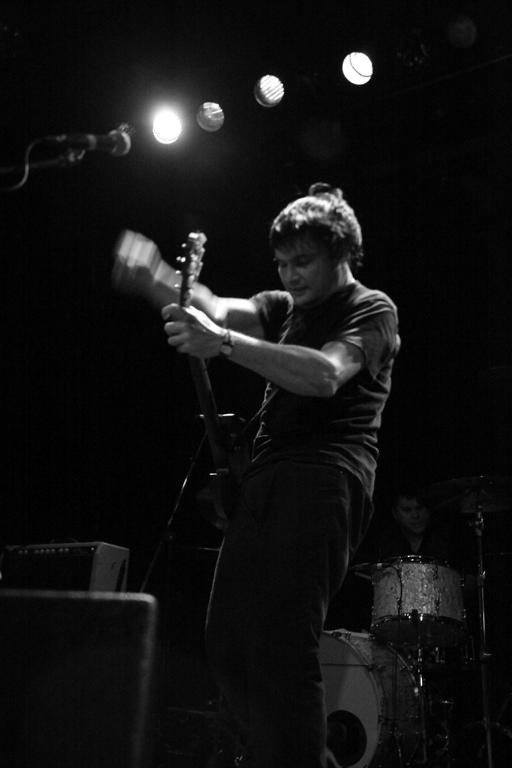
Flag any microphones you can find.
[349,562,392,572]
[326,722,348,745]
[40,127,133,159]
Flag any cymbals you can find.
[416,478,512,511]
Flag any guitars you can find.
[175,232,246,532]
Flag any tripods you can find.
[459,511,512,768]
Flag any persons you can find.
[112,182,404,748]
[377,483,458,569]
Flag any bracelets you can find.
[218,326,238,362]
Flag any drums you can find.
[371,555,471,641]
[319,629,431,766]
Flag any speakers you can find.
[0,588,156,768]
